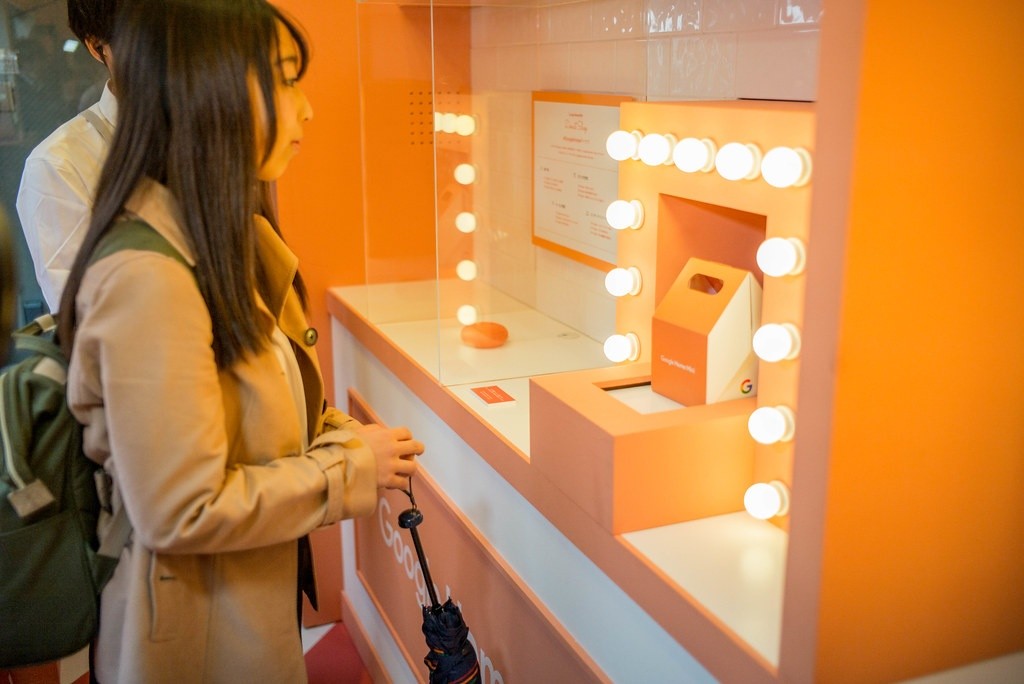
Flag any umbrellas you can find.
[398,510,482,684]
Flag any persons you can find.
[59,0,423,684]
[15,0,118,315]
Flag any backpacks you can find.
[0,224,211,668]
